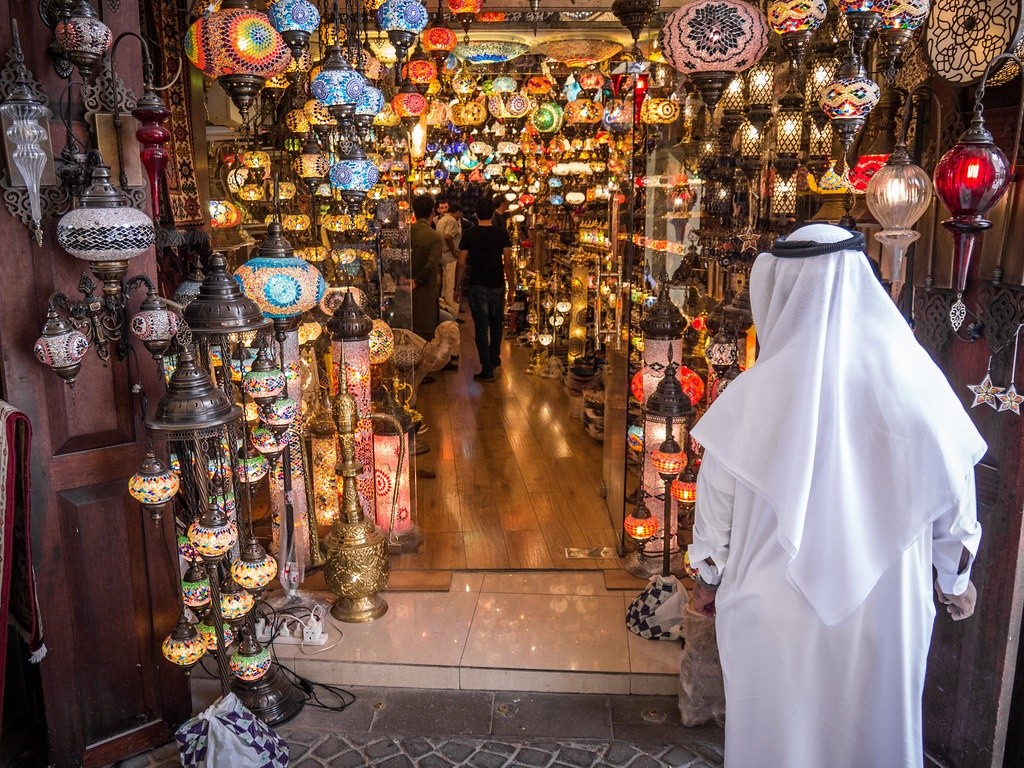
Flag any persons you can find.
[684,223,989,768]
[399,191,516,384]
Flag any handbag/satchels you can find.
[174,691,291,768]
[626,573,689,641]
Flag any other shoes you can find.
[420,376,436,384]
[456,319,466,324]
[474,366,495,382]
[491,357,501,369]
[459,309,466,313]
[451,354,459,360]
[442,362,459,370]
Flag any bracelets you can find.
[697,575,720,590]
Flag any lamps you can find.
[0,0,1024,729]
